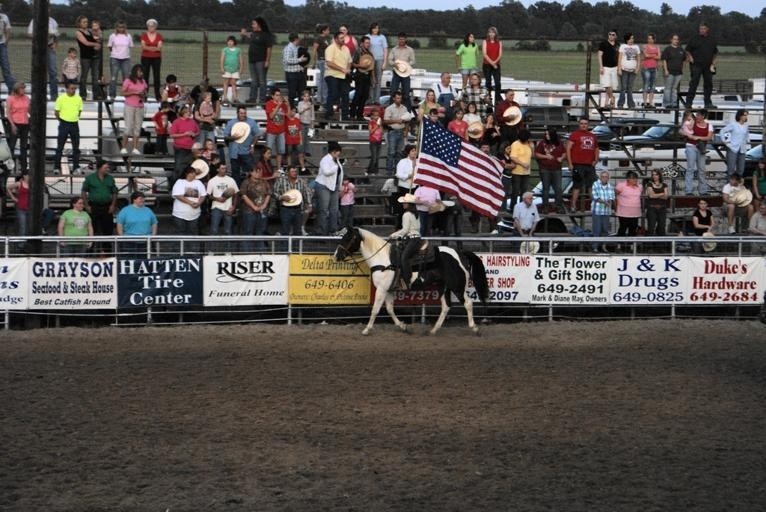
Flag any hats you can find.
[296,48,310,68]
[702,232,718,252]
[230,122,251,144]
[356,54,376,75]
[282,188,303,208]
[393,60,413,78]
[467,122,485,140]
[502,105,523,127]
[733,189,754,208]
[396,192,425,205]
[188,159,210,180]
[519,240,541,257]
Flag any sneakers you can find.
[245,98,267,103]
[131,148,142,155]
[72,168,84,177]
[119,147,129,155]
[53,168,60,176]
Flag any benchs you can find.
[0,86,766,255]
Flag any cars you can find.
[215,78,284,94]
[560,105,765,190]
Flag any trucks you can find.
[500,147,735,251]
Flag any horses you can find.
[332,222,490,338]
